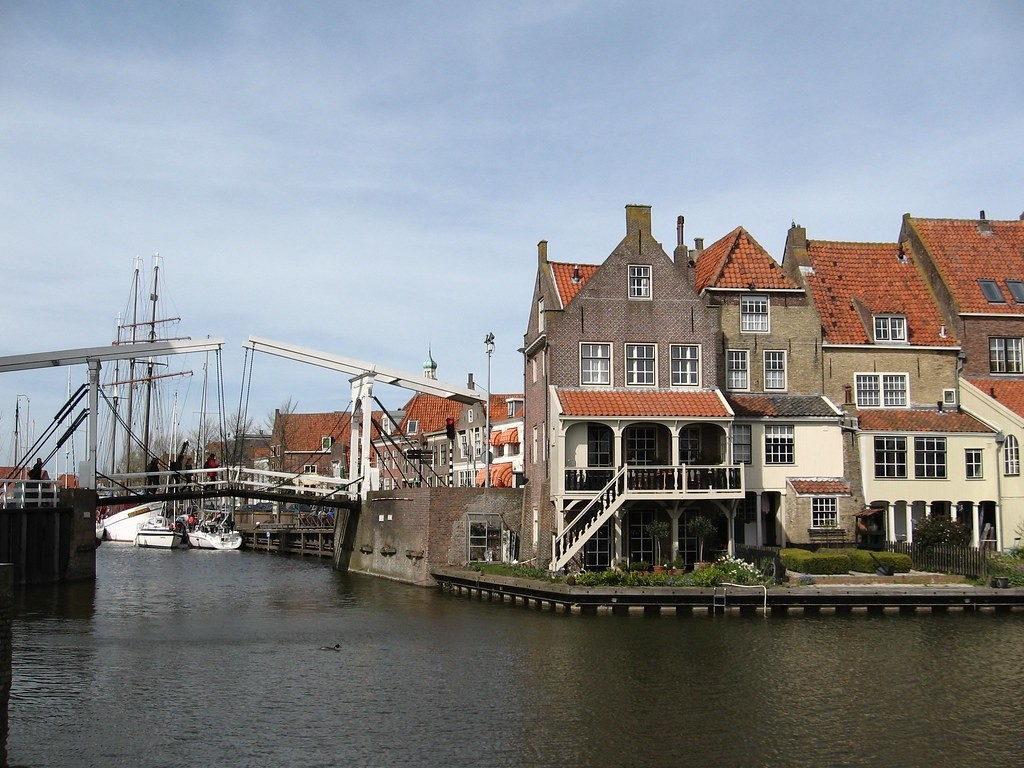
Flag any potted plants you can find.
[675,549,685,575]
[992,576,1010,588]
[688,516,716,570]
[630,562,640,577]
[646,520,675,575]
[640,561,650,576]
[667,561,674,576]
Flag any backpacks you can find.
[204,459,211,469]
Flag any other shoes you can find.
[149,491,152,494]
[180,489,183,492]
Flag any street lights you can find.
[484,332,495,488]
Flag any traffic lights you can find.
[446,418,455,439]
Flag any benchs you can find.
[807,528,848,549]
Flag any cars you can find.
[192,502,314,513]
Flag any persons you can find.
[318,510,334,527]
[145,453,222,495]
[33,458,43,479]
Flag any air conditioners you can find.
[512,461,525,473]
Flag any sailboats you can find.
[0,252,243,550]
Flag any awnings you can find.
[475,462,512,488]
[490,430,502,446]
[500,427,518,444]
